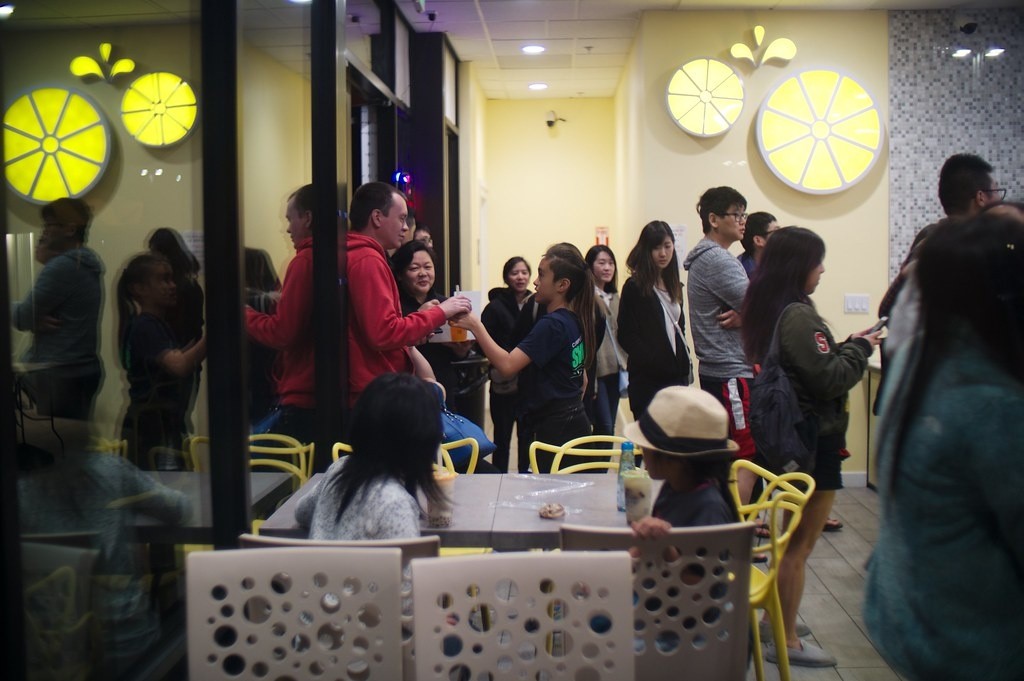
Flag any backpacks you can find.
[747,301,827,482]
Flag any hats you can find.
[622,385,740,456]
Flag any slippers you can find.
[757,523,774,538]
[823,519,843,531]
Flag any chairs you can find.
[183,432,814,681]
[11,435,210,681]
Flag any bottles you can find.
[616,441,637,512]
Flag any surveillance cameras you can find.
[427,10,437,20]
[951,11,979,34]
[546,111,555,127]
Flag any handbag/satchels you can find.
[618,370,629,398]
[689,358,694,385]
[433,383,498,469]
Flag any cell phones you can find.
[868,316,888,334]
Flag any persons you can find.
[876,151,1023,426]
[285,370,482,680]
[446,242,628,476]
[680,186,773,539]
[240,181,314,445]
[739,226,884,668]
[736,213,780,280]
[344,179,468,414]
[588,386,740,655]
[12,348,191,672]
[111,224,209,472]
[16,195,111,425]
[862,210,1024,681]
[616,220,695,423]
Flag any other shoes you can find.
[766,638,837,668]
[759,621,811,642]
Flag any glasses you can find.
[978,188,1006,200]
[714,212,748,222]
[416,238,433,243]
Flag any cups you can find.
[425,470,460,529]
[448,304,472,342]
[619,470,654,526]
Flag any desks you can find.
[489,472,667,551]
[259,473,507,546]
[17,468,294,642]
